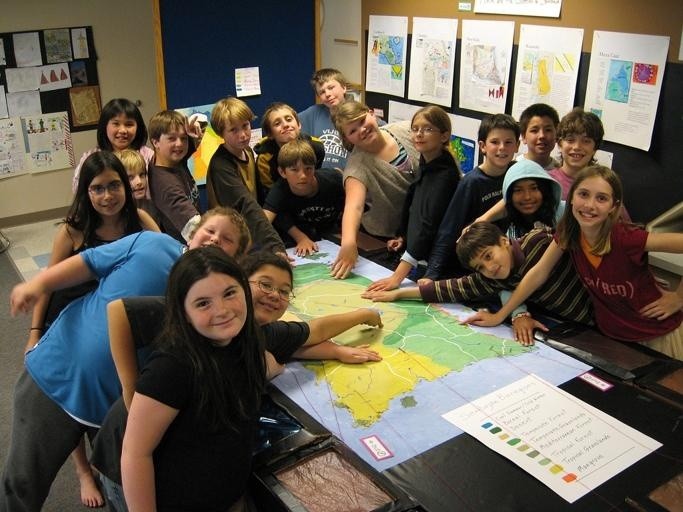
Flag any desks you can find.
[259,226,681,511]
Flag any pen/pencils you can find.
[625,497,647,512]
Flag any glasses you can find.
[408,127,446,136]
[246,279,296,303]
[86,181,123,196]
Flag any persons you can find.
[0,62,385,512]
[496,156,566,348]
[327,100,423,280]
[455,107,605,245]
[414,115,523,288]
[358,220,593,348]
[362,106,460,291]
[515,103,561,170]
[456,167,682,361]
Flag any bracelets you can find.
[512,311,531,322]
